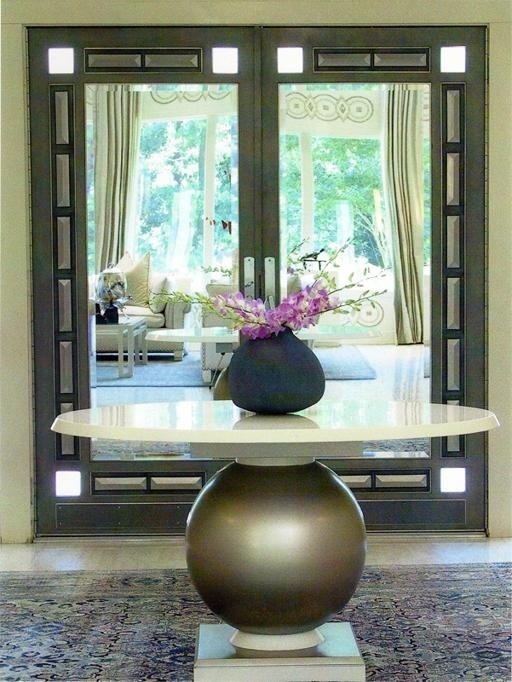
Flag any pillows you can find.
[115,251,172,314]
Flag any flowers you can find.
[150,234,389,341]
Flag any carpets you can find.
[0,563,512,681]
[97,344,376,387]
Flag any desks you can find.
[51,400,500,681]
[144,327,381,389]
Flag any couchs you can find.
[92,270,193,362]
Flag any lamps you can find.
[96,263,127,323]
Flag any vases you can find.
[229,331,325,414]
